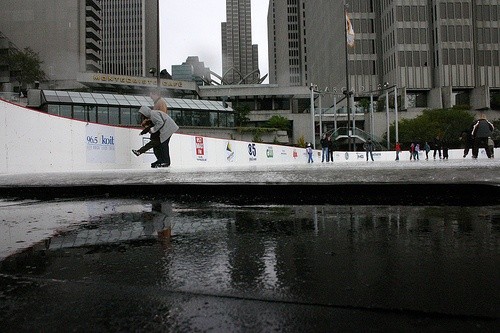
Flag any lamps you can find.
[149,68,156,76]
[34,81,40,89]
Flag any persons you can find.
[306,143,313,163]
[433,135,441,160]
[320,132,334,162]
[441,136,449,159]
[363,137,376,161]
[414,144,420,160]
[423,141,430,160]
[463,113,495,159]
[394,142,400,160]
[131,88,179,168]
[409,143,415,160]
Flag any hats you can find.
[308,143,311,146]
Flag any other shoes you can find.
[159,163,168,167]
[132,150,140,156]
